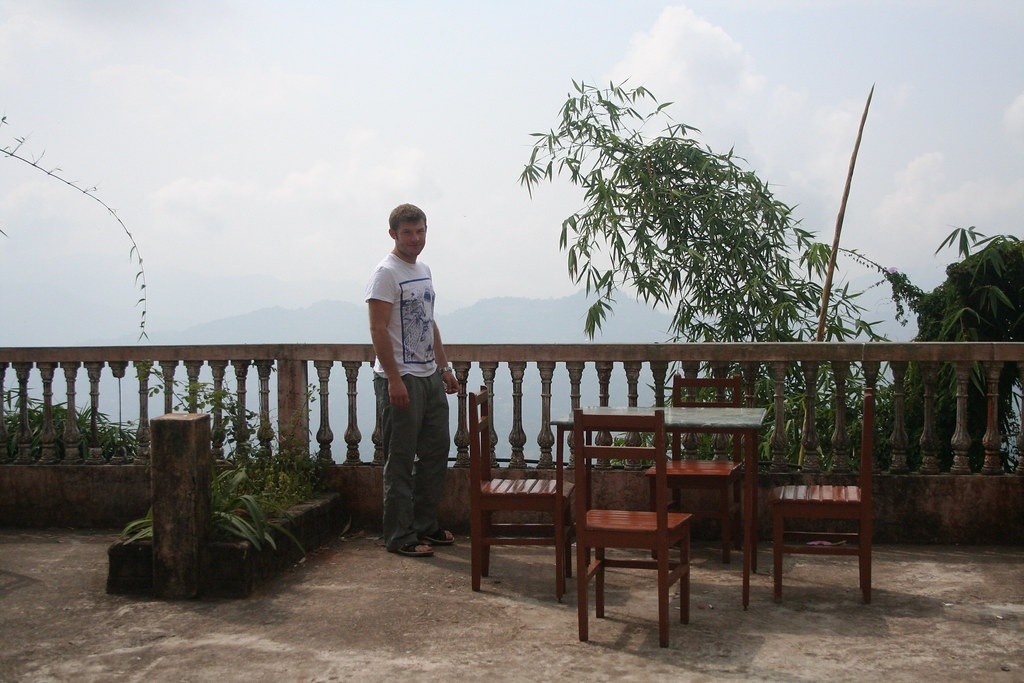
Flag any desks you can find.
[548,408,767,610]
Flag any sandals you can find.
[419,528,455,544]
[394,543,434,556]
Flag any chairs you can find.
[468,386,573,598]
[768,387,875,605]
[573,407,694,648]
[645,374,743,567]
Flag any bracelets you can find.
[439,367,452,375]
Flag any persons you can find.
[365,204,461,557]
